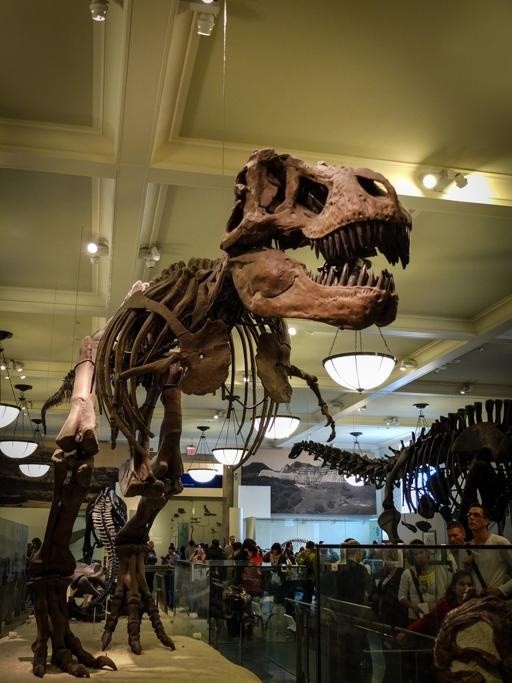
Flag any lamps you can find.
[186,394,303,483]
[138,243,161,270]
[0,330,51,479]
[342,431,369,487]
[320,326,398,399]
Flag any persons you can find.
[26,536,42,577]
